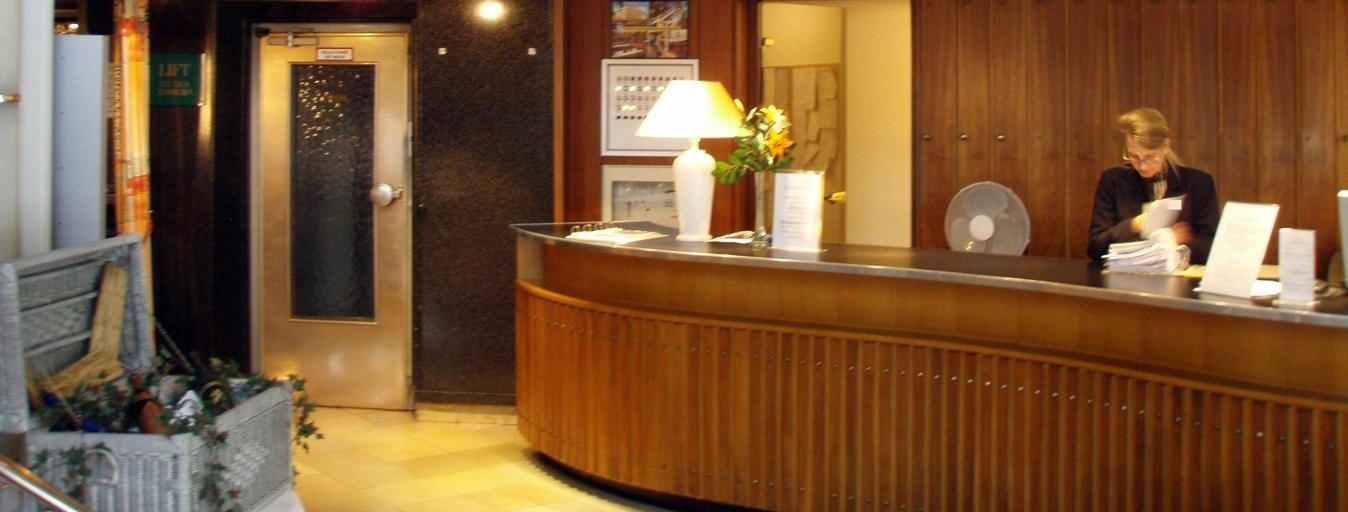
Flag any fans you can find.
[944,180,1031,258]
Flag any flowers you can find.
[713,98,846,205]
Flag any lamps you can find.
[634,77,756,239]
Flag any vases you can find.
[753,170,776,250]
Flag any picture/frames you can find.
[599,55,700,230]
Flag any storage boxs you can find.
[3,231,293,512]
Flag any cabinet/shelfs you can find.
[910,2,1345,262]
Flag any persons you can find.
[1084,106,1222,269]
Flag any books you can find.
[1100,226,1192,277]
